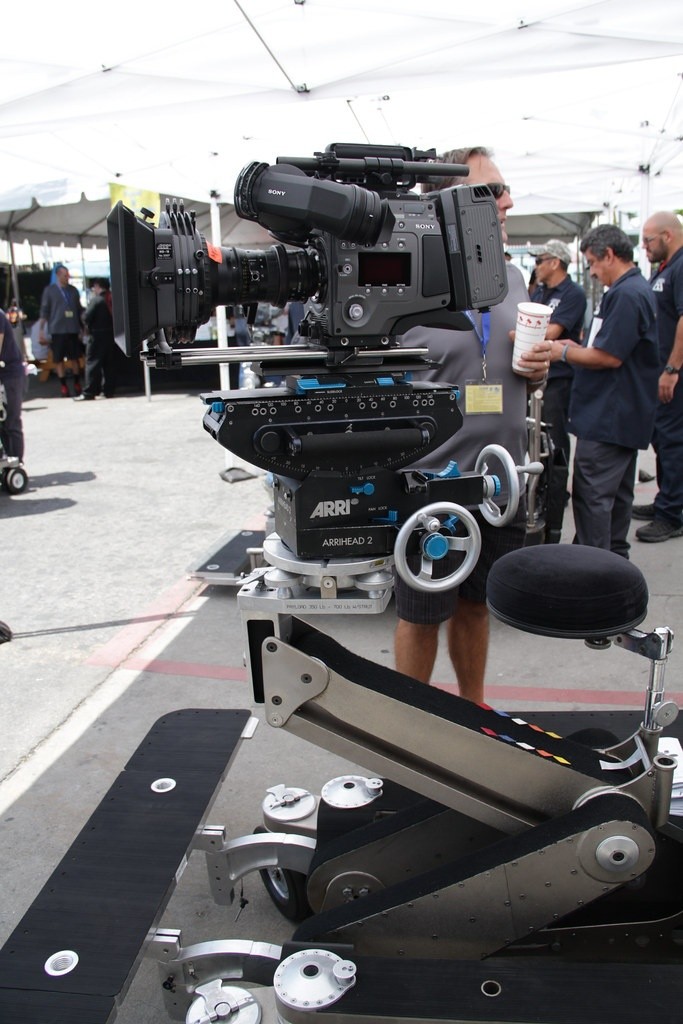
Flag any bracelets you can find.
[561,345,568,363]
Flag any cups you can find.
[512,301,553,372]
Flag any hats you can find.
[527,240,570,266]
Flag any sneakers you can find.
[631,504,655,519]
[636,519,683,542]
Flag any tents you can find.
[0,0,682,390]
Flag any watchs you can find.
[664,365,679,376]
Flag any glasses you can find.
[536,256,557,264]
[488,183,511,197]
[643,231,664,244]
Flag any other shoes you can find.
[62,385,68,397]
[74,394,94,400]
[95,392,113,399]
[73,384,81,395]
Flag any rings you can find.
[545,361,549,375]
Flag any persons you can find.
[553,224,659,560]
[630,211,683,543]
[31,265,122,401]
[0,309,25,462]
[5,298,27,333]
[526,238,591,544]
[397,145,553,704]
[272,297,312,395]
